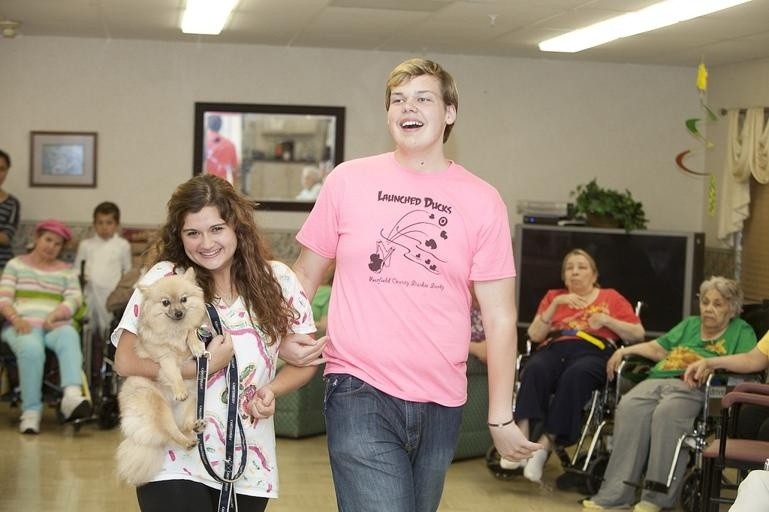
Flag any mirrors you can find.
[194,102,345,212]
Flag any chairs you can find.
[275,358,327,438]
[450,353,494,462]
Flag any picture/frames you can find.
[30,131,97,187]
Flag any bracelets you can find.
[488,418,514,428]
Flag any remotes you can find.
[556,220,586,226]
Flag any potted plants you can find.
[569,177,648,236]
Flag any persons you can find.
[276,259,335,372]
[0,148,20,401]
[499,250,646,482]
[74,202,132,398]
[0,219,90,433]
[278,58,545,512]
[106,233,167,312]
[583,276,757,512]
[204,114,240,196]
[109,174,319,512]
[468,281,487,366]
[684,329,769,388]
[295,166,323,200]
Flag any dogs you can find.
[115,266,207,488]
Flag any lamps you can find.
[539,1,745,53]
[180,1,239,35]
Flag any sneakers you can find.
[60,394,92,418]
[524,448,548,482]
[583,500,631,511]
[633,501,662,512]
[500,456,521,470]
[19,411,40,435]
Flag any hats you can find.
[35,220,72,241]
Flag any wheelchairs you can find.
[0,259,124,430]
